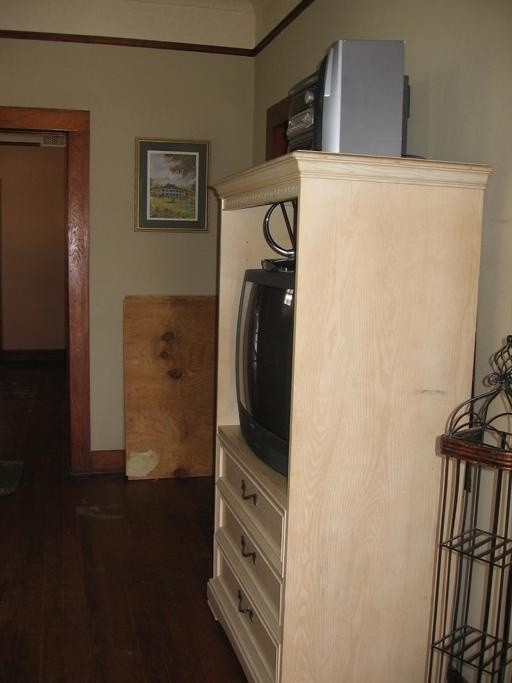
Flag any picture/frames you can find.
[131,134,212,234]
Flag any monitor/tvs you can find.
[235,268,295,480]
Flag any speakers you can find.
[312,39,404,158]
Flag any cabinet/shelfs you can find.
[205,149,492,681]
